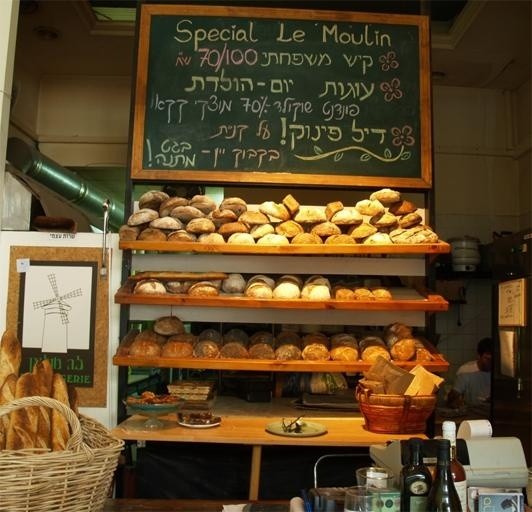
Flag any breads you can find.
[331,338,358,361]
[137,228,174,241]
[389,223,439,244]
[170,205,207,225]
[358,334,386,350]
[222,274,246,293]
[133,279,167,295]
[238,211,271,225]
[51,374,71,452]
[325,201,344,221]
[331,332,358,348]
[350,286,375,301]
[370,188,401,204]
[67,384,79,437]
[5,373,40,456]
[388,198,418,216]
[293,208,327,225]
[148,216,186,230]
[164,282,193,294]
[153,316,185,335]
[139,190,170,213]
[167,229,197,242]
[160,342,195,359]
[282,194,300,216]
[197,330,225,349]
[413,336,436,361]
[304,275,333,294]
[204,208,238,223]
[292,232,324,244]
[310,222,342,237]
[118,224,145,240]
[188,195,216,215]
[325,234,357,245]
[258,200,291,221]
[331,208,364,225]
[277,331,304,351]
[302,344,331,361]
[127,208,159,226]
[185,218,216,234]
[32,360,53,455]
[159,197,189,218]
[369,286,393,301]
[361,232,394,246]
[256,234,290,245]
[128,339,160,358]
[167,334,198,348]
[187,280,222,296]
[193,341,220,361]
[248,344,275,360]
[246,333,282,354]
[275,220,304,238]
[302,333,331,352]
[245,274,275,290]
[215,343,250,360]
[196,232,226,244]
[134,330,166,348]
[301,283,331,300]
[384,322,415,361]
[249,224,275,239]
[223,330,249,352]
[272,278,301,300]
[355,199,385,216]
[398,213,423,229]
[217,221,251,237]
[219,197,247,217]
[127,272,230,281]
[347,223,378,238]
[244,279,273,299]
[276,275,303,288]
[0,327,21,391]
[332,285,356,301]
[0,374,18,450]
[227,232,256,245]
[369,212,398,227]
[275,344,304,360]
[360,341,391,363]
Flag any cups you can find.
[356,468,395,489]
[344,486,381,512]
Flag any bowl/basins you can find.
[122,395,185,428]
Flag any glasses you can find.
[281,412,306,432]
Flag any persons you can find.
[446,338,492,409]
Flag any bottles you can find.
[429,439,463,512]
[400,438,434,512]
[441,421,467,512]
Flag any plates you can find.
[265,421,326,437]
[177,417,222,429]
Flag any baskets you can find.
[1,395,125,512]
[356,387,437,434]
[167,379,215,400]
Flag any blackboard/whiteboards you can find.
[128,3,432,190]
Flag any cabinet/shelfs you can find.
[112,204,450,500]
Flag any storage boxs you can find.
[500,331,531,379]
[498,278,528,327]
